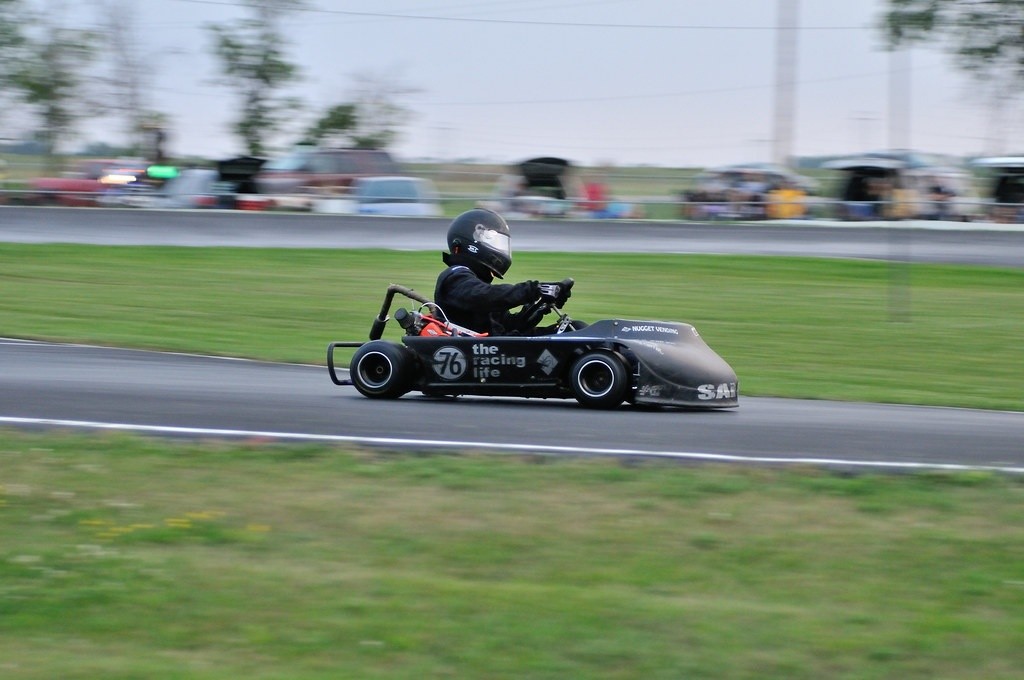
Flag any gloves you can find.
[536,280,572,309]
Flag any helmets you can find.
[447,208,512,279]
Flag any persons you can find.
[845,172,879,202]
[236,172,259,194]
[433,208,588,338]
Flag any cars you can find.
[153,168,233,212]
[685,167,820,222]
[970,159,1024,206]
[254,147,395,196]
[484,157,592,221]
[350,176,442,223]
[822,158,908,221]
[33,157,155,206]
